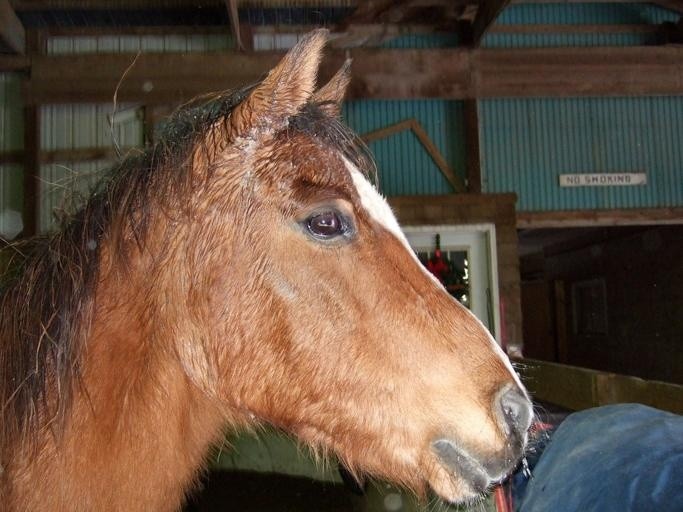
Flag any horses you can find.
[0,25,555,512]
